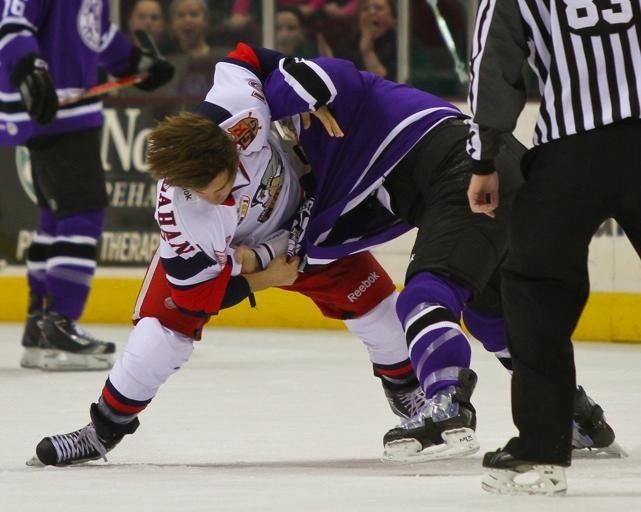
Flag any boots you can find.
[37,394,139,463]
[572,384,614,448]
[382,368,478,447]
[21,290,114,354]
[379,372,428,421]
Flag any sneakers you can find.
[482,450,535,467]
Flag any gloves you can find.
[10,52,58,124]
[113,45,174,91]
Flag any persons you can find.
[467,1,641,469]
[225,51,615,452]
[0,2,176,357]
[127,0,399,100]
[36,36,430,469]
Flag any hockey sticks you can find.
[55,28,166,105]
